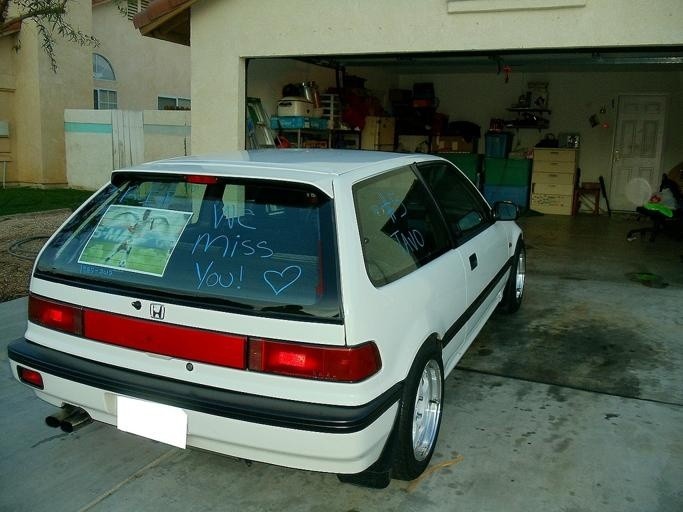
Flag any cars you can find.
[7,147,528,490]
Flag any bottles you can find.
[301,80,321,108]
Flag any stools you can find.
[574,188,600,216]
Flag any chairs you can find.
[626,178,681,244]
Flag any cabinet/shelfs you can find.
[530,147,577,215]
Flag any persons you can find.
[102,208,152,268]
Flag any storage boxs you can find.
[483,130,531,214]
[433,136,480,184]
